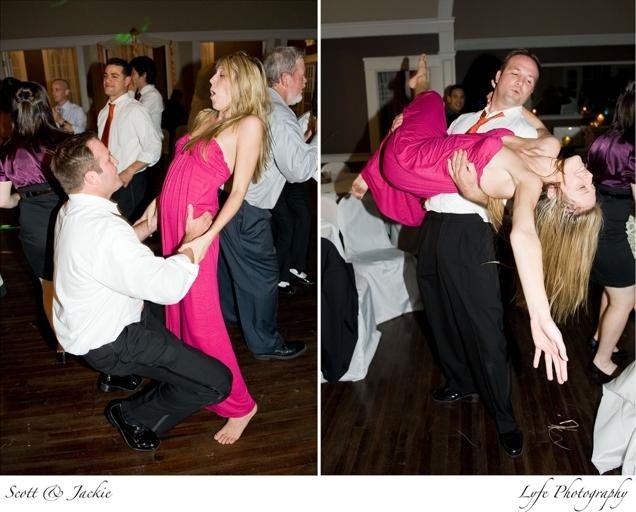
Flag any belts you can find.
[21,187,55,199]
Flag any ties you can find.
[101,102,116,149]
[464,109,504,135]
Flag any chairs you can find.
[321,158,353,177]
[323,189,383,385]
[336,193,426,327]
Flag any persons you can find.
[0,44,318,452]
[334,45,636,461]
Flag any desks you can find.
[319,171,381,218]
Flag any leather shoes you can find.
[588,361,617,384]
[253,336,306,363]
[432,383,481,405]
[103,397,162,453]
[497,428,526,456]
[589,337,628,361]
[100,372,146,393]
